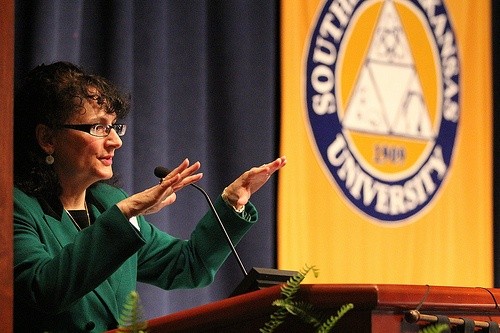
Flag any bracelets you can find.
[223,186,245,212]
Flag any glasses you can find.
[48,124,127,137]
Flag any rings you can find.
[159,177,164,183]
[263,163,267,166]
[266,173,268,175]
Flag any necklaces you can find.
[62,200,90,231]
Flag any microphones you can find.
[153,166,305,298]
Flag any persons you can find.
[13,62,288,333]
